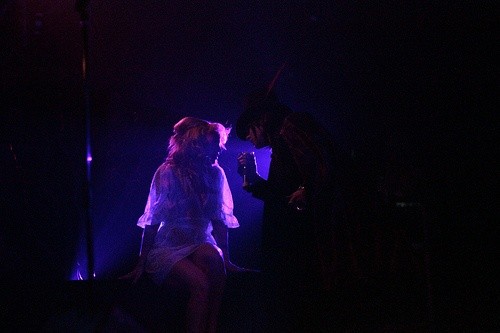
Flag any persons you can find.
[235,90,379,333]
[117,117,257,333]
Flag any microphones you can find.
[242,165,248,188]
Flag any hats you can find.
[235,90,291,139]
